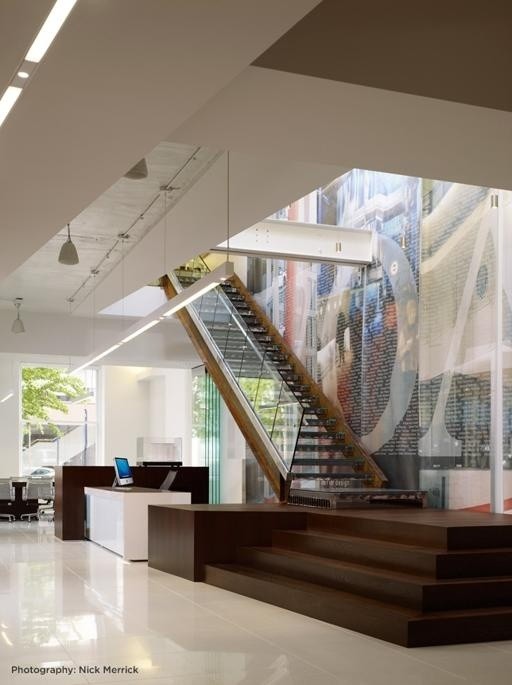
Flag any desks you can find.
[83,486,192,562]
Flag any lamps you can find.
[124,156,148,179]
[58,223,79,265]
[11,298,25,334]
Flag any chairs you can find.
[0,476,55,522]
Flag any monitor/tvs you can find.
[111,457,134,489]
